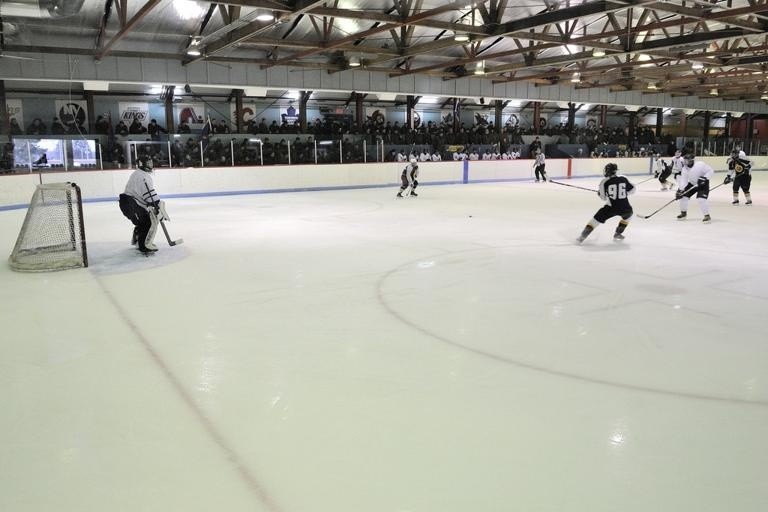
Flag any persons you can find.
[533,149,546,182]
[652,152,674,191]
[575,163,635,246]
[397,158,420,196]
[119,154,168,255]
[669,151,686,183]
[726,150,753,206]
[676,153,713,221]
[725,145,746,182]
[6,108,693,163]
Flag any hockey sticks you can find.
[143,178,183,246]
[549,178,599,192]
[636,184,697,219]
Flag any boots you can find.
[613,231,627,239]
[732,197,740,204]
[660,183,676,191]
[410,191,418,196]
[576,235,586,243]
[397,193,403,197]
[132,237,159,254]
[703,215,711,221]
[745,196,753,205]
[677,211,687,218]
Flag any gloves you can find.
[697,176,708,189]
[724,174,732,184]
[654,172,659,179]
[675,189,684,200]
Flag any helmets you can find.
[604,163,618,176]
[410,158,417,166]
[135,154,153,171]
[654,144,743,165]
